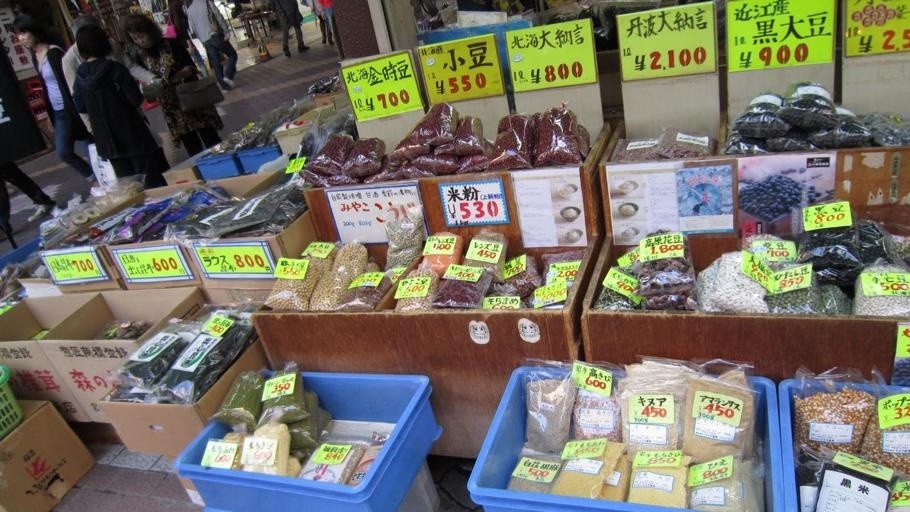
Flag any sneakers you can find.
[223,77,237,89]
[28,204,50,222]
[283,39,335,58]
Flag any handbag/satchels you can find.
[208,12,228,33]
[177,78,224,111]
[162,16,178,39]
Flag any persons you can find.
[0,93,57,239]
[120,12,226,157]
[269,0,310,58]
[9,14,103,187]
[180,0,239,94]
[310,0,338,46]
[52,13,166,144]
[67,22,172,191]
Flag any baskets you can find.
[0,365,23,440]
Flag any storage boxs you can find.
[178,369,444,510]
[0,291,101,428]
[1,364,21,432]
[106,171,274,283]
[2,399,99,512]
[188,184,320,305]
[466,366,786,512]
[101,300,268,456]
[39,177,203,282]
[778,377,910,512]
[40,286,205,427]
[164,91,350,185]
